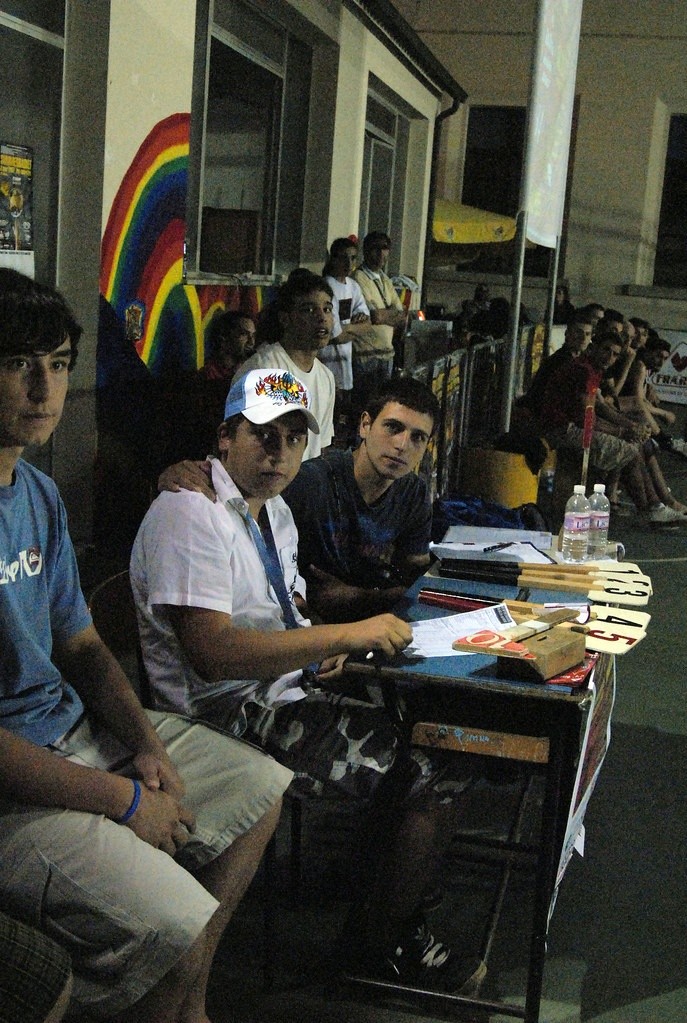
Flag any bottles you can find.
[588,484,610,560]
[562,485,591,564]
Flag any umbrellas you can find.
[433,195,528,434]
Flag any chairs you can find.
[85,571,342,993]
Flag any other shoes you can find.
[648,505,687,530]
[659,435,687,455]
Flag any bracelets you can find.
[113,779,141,824]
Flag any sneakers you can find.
[344,916,487,998]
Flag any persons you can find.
[456,283,535,341]
[129,268,488,997]
[191,231,404,431]
[0,267,295,1023]
[525,285,687,527]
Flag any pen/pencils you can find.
[483,542,515,552]
[367,650,381,659]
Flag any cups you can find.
[593,541,626,564]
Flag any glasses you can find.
[371,244,390,251]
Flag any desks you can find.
[342,524,627,1022]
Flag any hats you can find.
[223,368,320,435]
